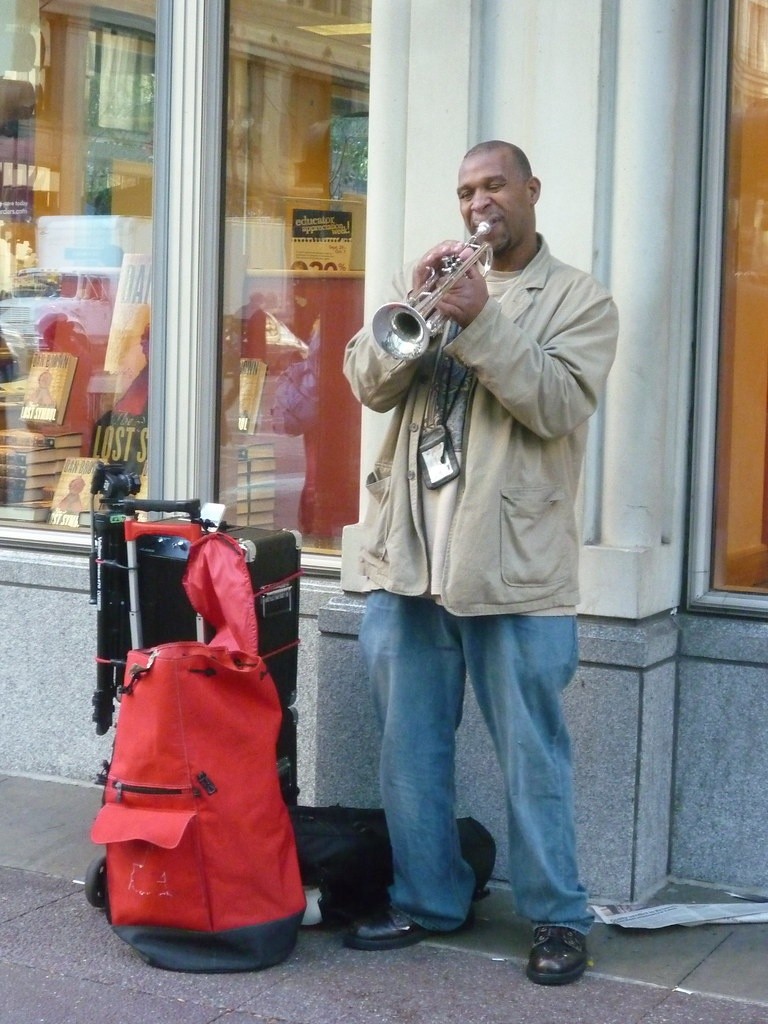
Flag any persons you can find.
[343,139,619,985]
[221,294,268,415]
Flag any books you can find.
[0,352,108,529]
[240,358,267,436]
[90,254,152,524]
[230,443,274,531]
[289,206,352,270]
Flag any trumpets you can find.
[372,221,493,362]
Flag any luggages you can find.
[79,513,308,973]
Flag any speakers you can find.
[126,516,301,810]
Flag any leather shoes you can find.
[529,928,588,987]
[344,903,466,951]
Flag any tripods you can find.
[88,459,142,735]
